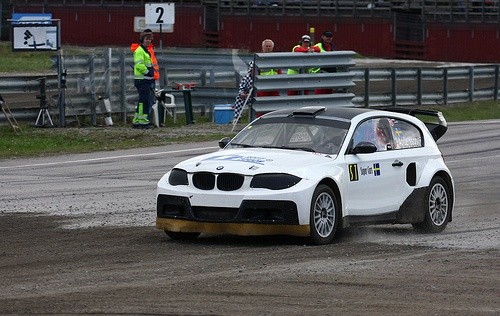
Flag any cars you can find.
[155,105,456,246]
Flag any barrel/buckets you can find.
[214,103,236,125]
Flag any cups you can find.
[175,82,196,91]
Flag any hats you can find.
[323,30,334,37]
[299,35,311,47]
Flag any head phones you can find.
[139,29,154,43]
[299,36,312,46]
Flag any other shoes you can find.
[145,126,154,129]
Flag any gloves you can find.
[145,70,154,77]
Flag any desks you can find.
[159,89,198,127]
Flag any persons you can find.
[232,31,337,127]
[130,29,159,129]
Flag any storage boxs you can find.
[214,107,234,124]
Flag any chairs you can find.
[154,88,176,128]
